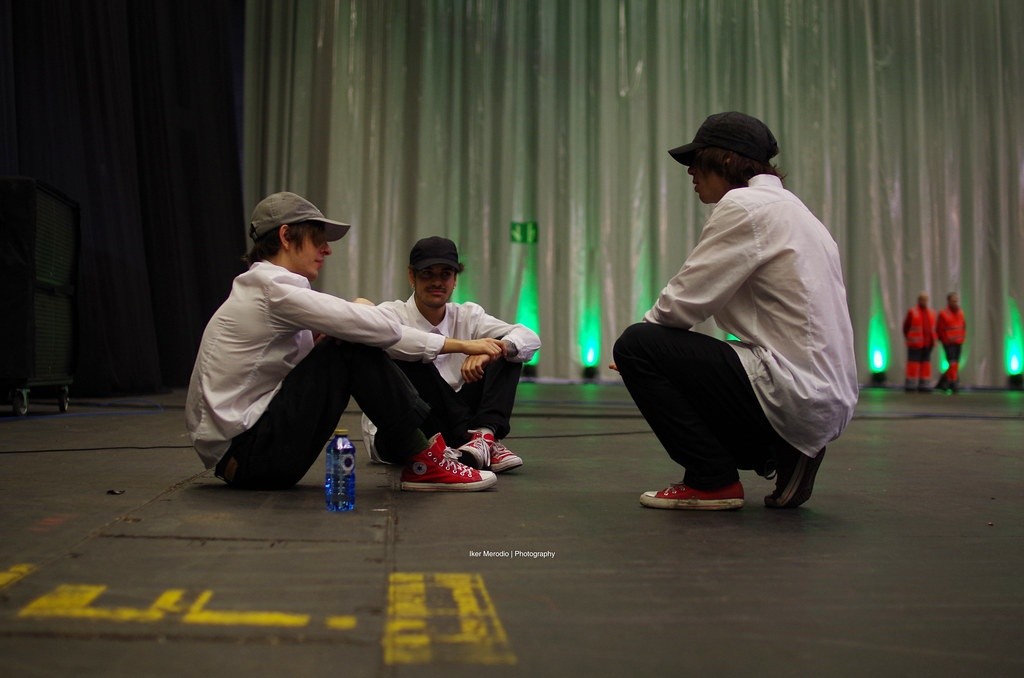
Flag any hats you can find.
[409,236,460,272]
[249,190,351,242]
[668,110,778,166]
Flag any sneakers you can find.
[400,432,498,493]
[457,431,523,471]
[639,480,745,510]
[763,441,827,508]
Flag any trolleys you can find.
[0,371,73,415]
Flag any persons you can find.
[937,292,966,394]
[609,112,859,511]
[185,192,497,492]
[361,237,541,473]
[903,292,937,393]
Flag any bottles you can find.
[325,429,356,512]
